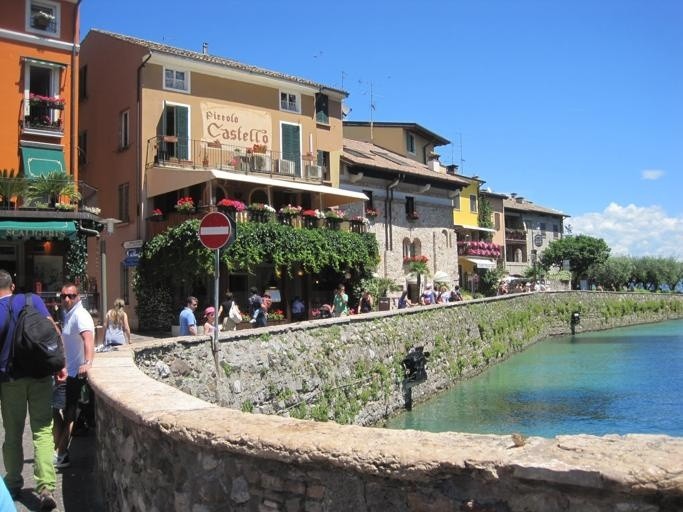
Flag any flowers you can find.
[403,255,429,264]
[148,197,370,228]
[458,241,502,258]
[29,92,66,107]
[31,116,64,130]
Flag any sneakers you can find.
[40,489,56,511]
[52,452,70,468]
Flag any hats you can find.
[203,307,214,317]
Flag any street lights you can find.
[96,217,123,329]
[531,219,541,285]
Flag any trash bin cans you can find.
[379,298,390,311]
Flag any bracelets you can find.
[83,358,93,366]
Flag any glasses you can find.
[61,294,76,300]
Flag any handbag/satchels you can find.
[228,300,242,325]
[68,375,97,473]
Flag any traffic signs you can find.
[124,248,142,257]
[121,238,144,248]
[120,257,140,266]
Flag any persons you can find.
[498,281,546,295]
[248,286,281,328]
[0,270,67,511]
[51,281,96,468]
[179,295,199,335]
[202,305,219,335]
[218,290,241,332]
[399,286,462,309]
[101,296,133,348]
[292,284,373,322]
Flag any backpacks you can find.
[5,293,65,380]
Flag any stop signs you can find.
[196,211,233,251]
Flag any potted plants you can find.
[0,168,81,211]
